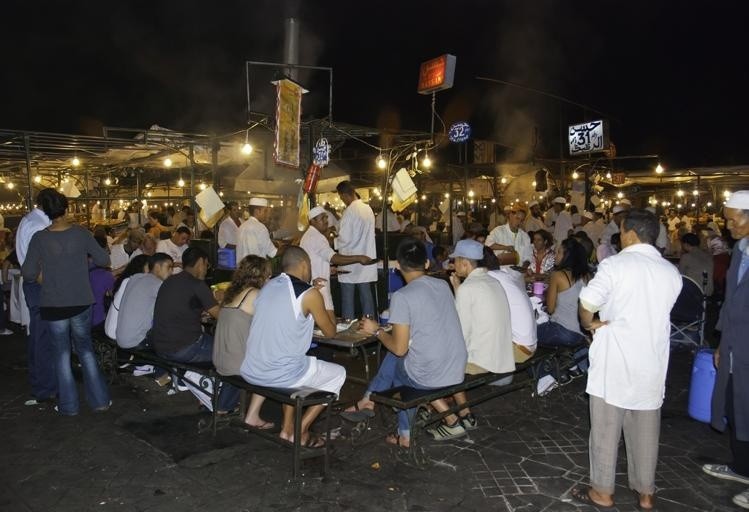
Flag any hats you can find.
[508,196,625,221]
[725,190,749,212]
[449,239,485,260]
[308,205,327,221]
[248,197,268,207]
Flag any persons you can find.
[570,208,686,512]
[329,181,376,318]
[701,189,749,508]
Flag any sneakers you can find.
[458,413,480,431]
[732,488,749,509]
[115,353,244,416]
[702,463,749,484]
[530,361,584,404]
[426,417,468,441]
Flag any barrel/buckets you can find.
[688,349,718,423]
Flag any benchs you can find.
[90,325,336,478]
[370,332,593,469]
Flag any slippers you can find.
[339,401,376,422]
[571,487,614,509]
[638,493,656,511]
[385,433,415,449]
[278,431,331,448]
[246,421,275,430]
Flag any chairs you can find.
[659,263,715,358]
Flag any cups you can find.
[363,313,373,320]
[532,282,548,295]
[496,249,518,266]
